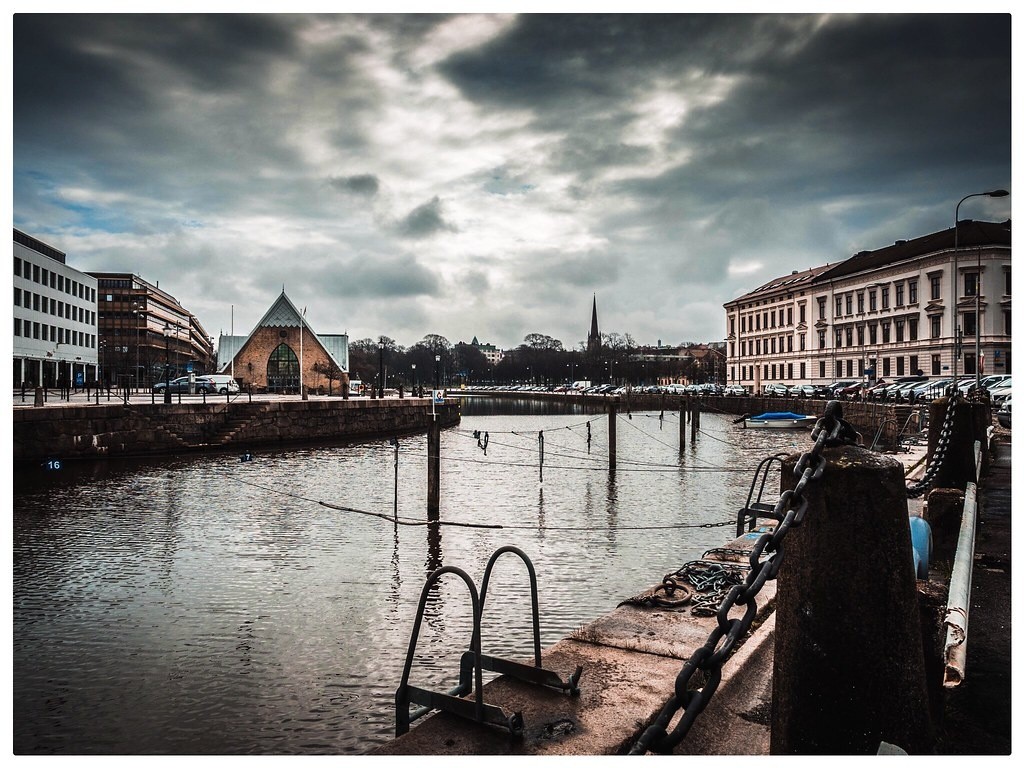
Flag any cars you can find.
[200,374,240,395]
[723,384,750,396]
[765,383,788,397]
[153,376,217,394]
[578,382,725,396]
[787,385,814,398]
[817,373,1012,429]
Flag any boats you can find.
[732,411,817,429]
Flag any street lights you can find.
[162,320,171,404]
[435,353,441,389]
[953,190,1010,382]
[378,337,385,398]
[411,362,417,396]
